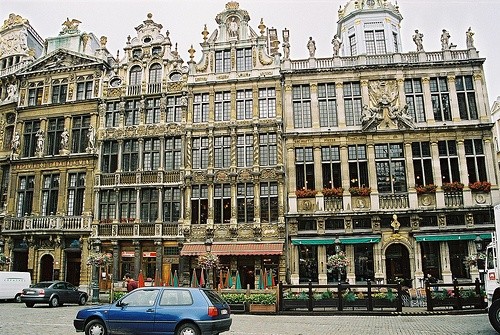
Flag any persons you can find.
[11,132,21,149]
[36,129,45,151]
[87,125,96,148]
[60,128,70,149]
[124,273,138,292]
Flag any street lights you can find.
[91,238,102,305]
[333,239,342,299]
[204,238,213,289]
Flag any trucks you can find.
[477,204,500,335]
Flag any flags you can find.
[225,269,232,288]
[154,269,179,287]
[191,268,198,287]
[138,270,144,287]
[220,269,223,288]
[200,268,206,287]
[258,268,273,288]
[235,274,241,289]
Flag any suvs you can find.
[0,271,31,303]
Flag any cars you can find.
[73,286,232,335]
[20,280,89,308]
